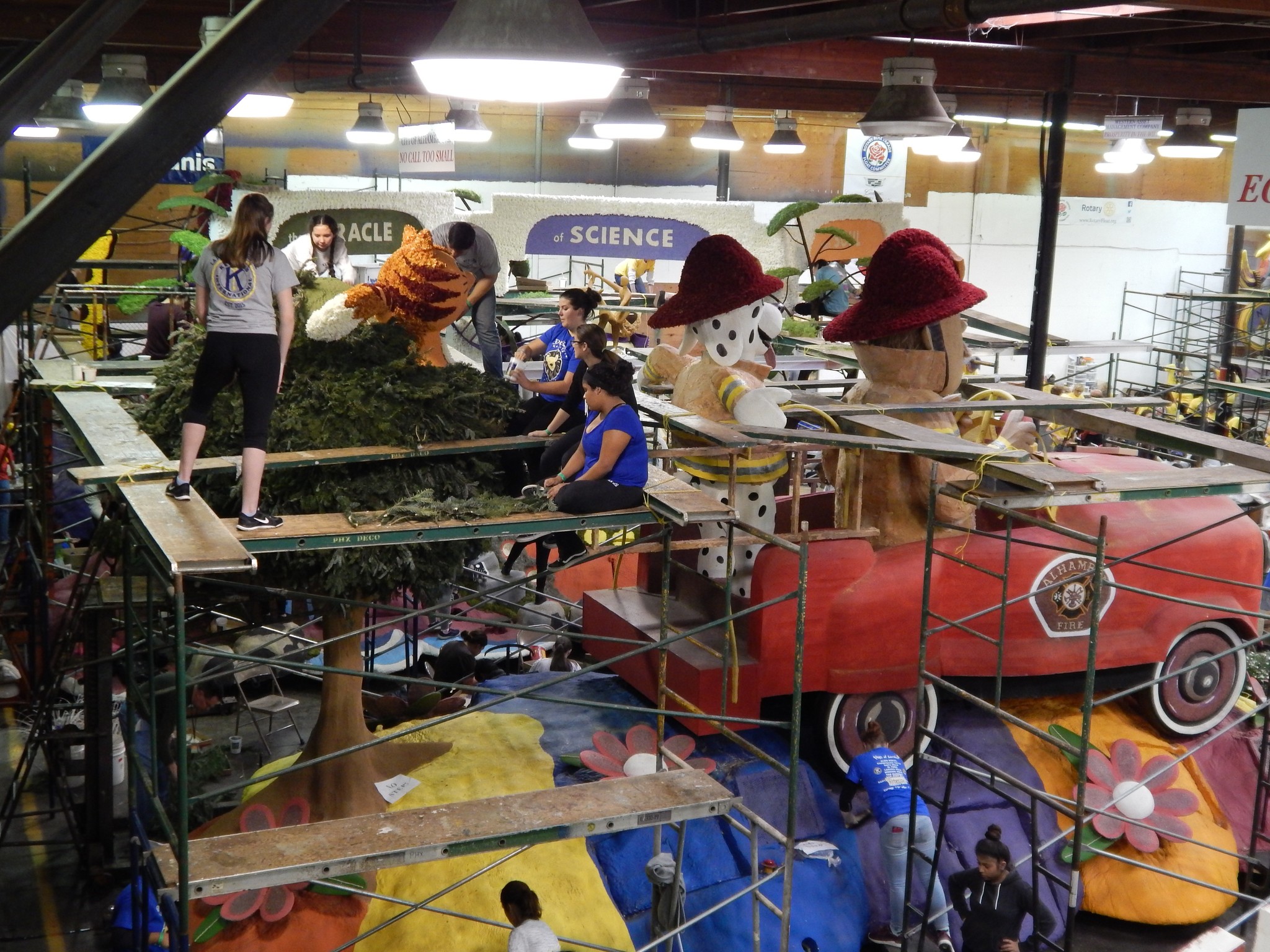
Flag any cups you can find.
[137,355,151,361]
[71,365,88,381]
[82,368,97,382]
[504,356,527,383]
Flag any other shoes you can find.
[869,925,902,948]
[938,932,955,952]
[501,564,512,575]
[535,596,547,604]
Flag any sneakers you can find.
[547,547,590,572]
[428,620,452,629]
[542,535,558,549]
[236,507,284,531]
[438,628,460,639]
[522,485,545,497]
[166,476,191,500]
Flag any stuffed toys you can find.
[822,228,1036,555]
[304,224,476,369]
[636,233,792,599]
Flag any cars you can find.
[579,379,1267,784]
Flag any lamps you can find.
[762,110,806,154]
[593,69,667,140]
[441,98,493,143]
[568,99,615,152]
[1092,98,1224,175]
[691,81,744,152]
[345,91,395,145]
[410,1,626,103]
[857,33,981,162]
[13,1,293,139]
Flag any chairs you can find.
[233,658,306,756]
[517,624,558,675]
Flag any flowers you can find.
[192,798,367,944]
[559,725,717,781]
[361,677,466,731]
[1048,724,1199,863]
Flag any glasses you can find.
[570,339,585,347]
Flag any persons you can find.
[282,213,356,285]
[828,258,863,305]
[50,270,81,329]
[794,258,849,317]
[142,193,299,532]
[612,258,655,293]
[432,220,505,379]
[1042,354,1270,469]
[838,721,955,952]
[947,824,1057,952]
[963,354,982,375]
[511,287,649,572]
[97,339,125,361]
[0,445,582,952]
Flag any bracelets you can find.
[465,300,472,310]
[544,429,552,436]
[557,473,567,482]
[65,537,68,542]
[157,932,164,946]
